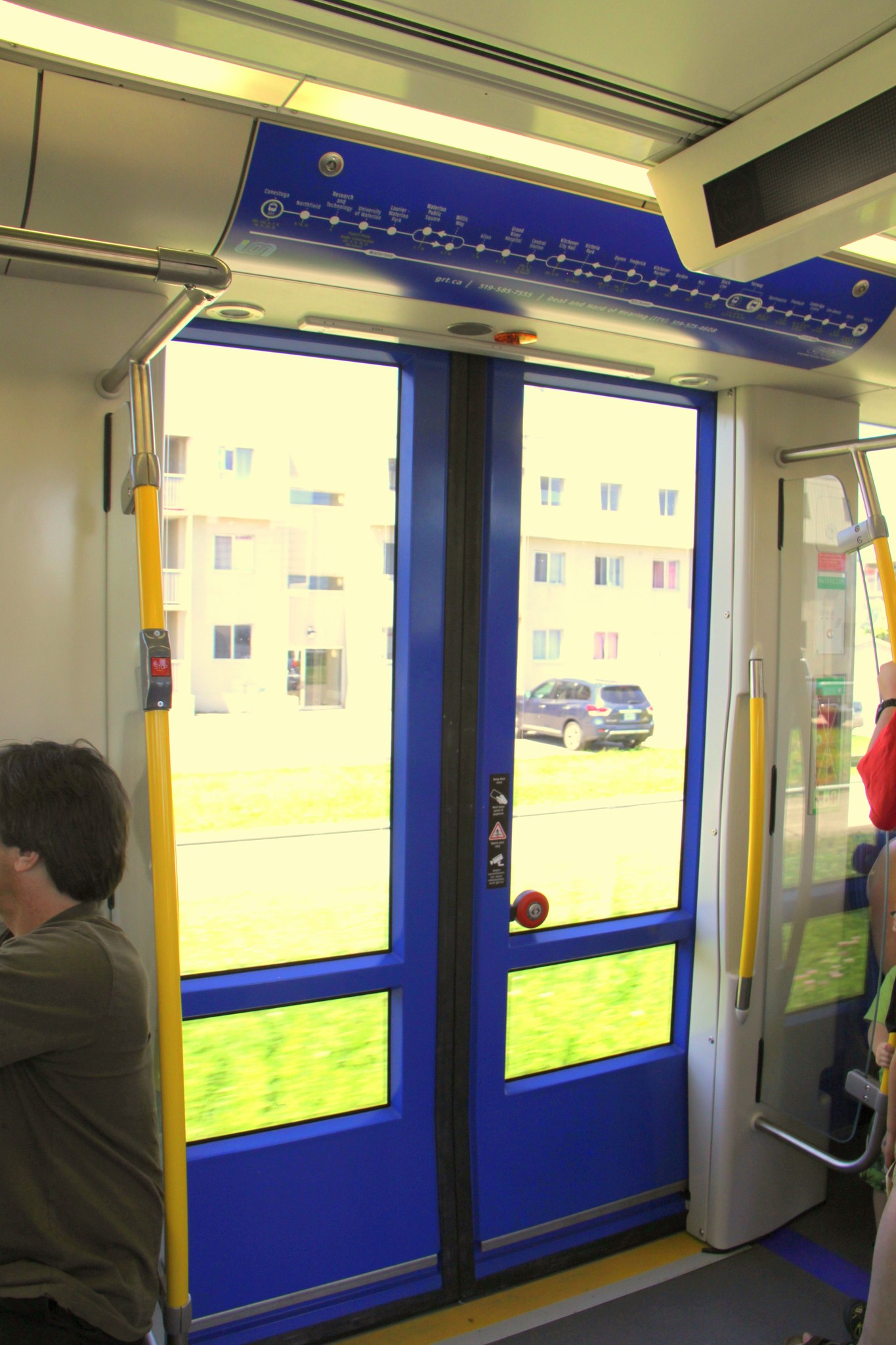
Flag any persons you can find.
[0,736,164,1345]
[785,660,896,1345]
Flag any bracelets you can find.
[875,699,896,724]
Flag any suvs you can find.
[515,677,655,751]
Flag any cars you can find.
[288,659,301,692]
